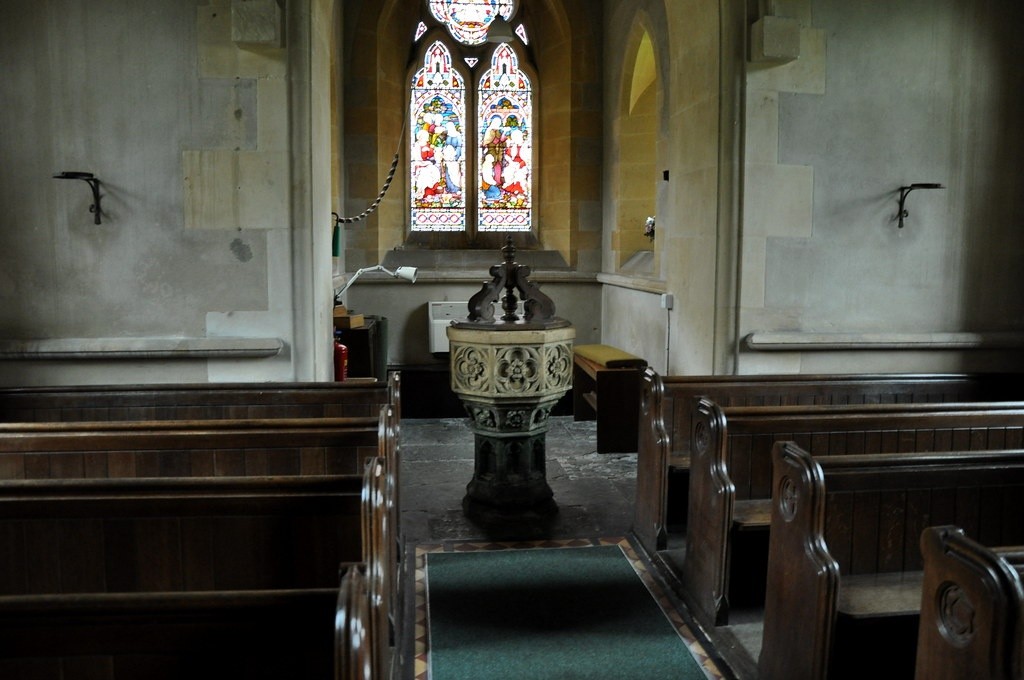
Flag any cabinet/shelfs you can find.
[337,319,377,378]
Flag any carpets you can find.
[411,527,722,680]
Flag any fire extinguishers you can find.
[332,330,347,383]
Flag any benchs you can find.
[682,392,1024,624]
[632,364,1024,550]
[571,340,651,453]
[2,371,405,679]
[755,440,1024,679]
